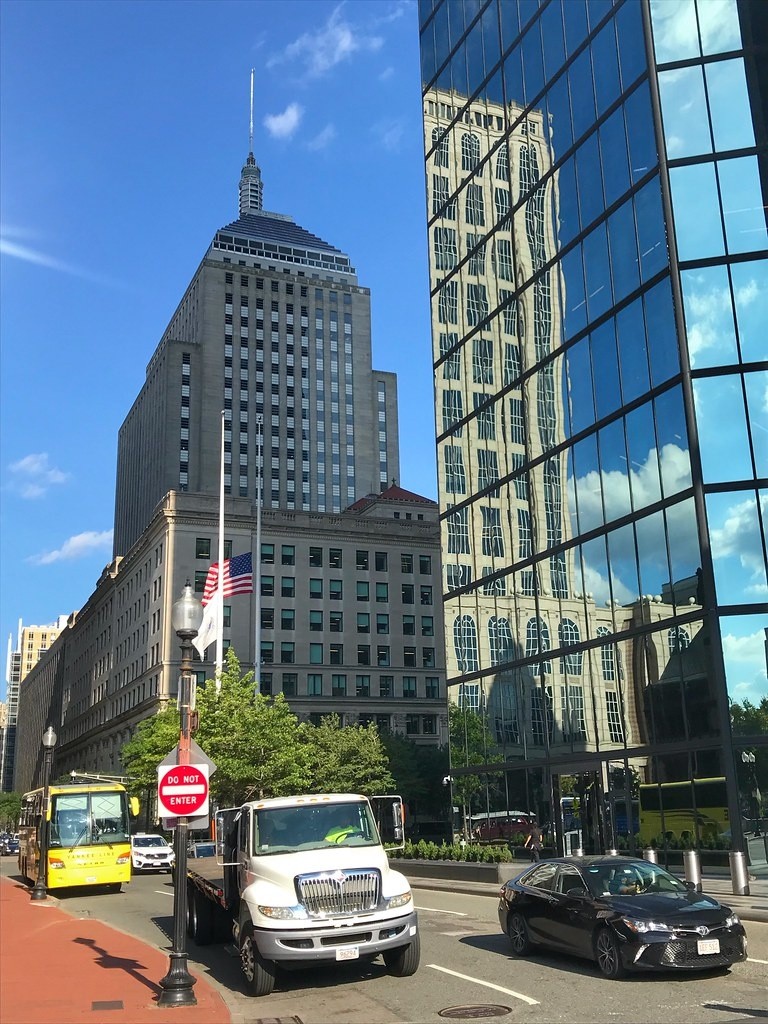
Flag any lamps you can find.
[442,774,451,787]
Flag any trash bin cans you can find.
[606,849,620,879]
[643,850,660,886]
[572,848,586,857]
[729,852,751,895]
[682,851,703,892]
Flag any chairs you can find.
[558,875,585,896]
[605,867,624,893]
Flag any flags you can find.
[202,552,253,607]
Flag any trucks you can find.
[170,793,420,997]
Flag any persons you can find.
[149,840,158,847]
[322,815,360,843]
[619,874,640,894]
[524,822,543,862]
[473,826,482,846]
[98,820,116,834]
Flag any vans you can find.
[474,815,530,841]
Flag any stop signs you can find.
[156,763,209,818]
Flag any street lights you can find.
[30,724,58,905]
[741,751,762,837]
[154,576,205,1006]
[441,774,455,847]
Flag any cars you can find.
[497,855,748,980]
[127,832,176,875]
[0,832,19,856]
[187,842,225,859]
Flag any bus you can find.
[18,782,140,893]
[560,796,586,833]
[604,799,639,837]
[638,777,733,850]
